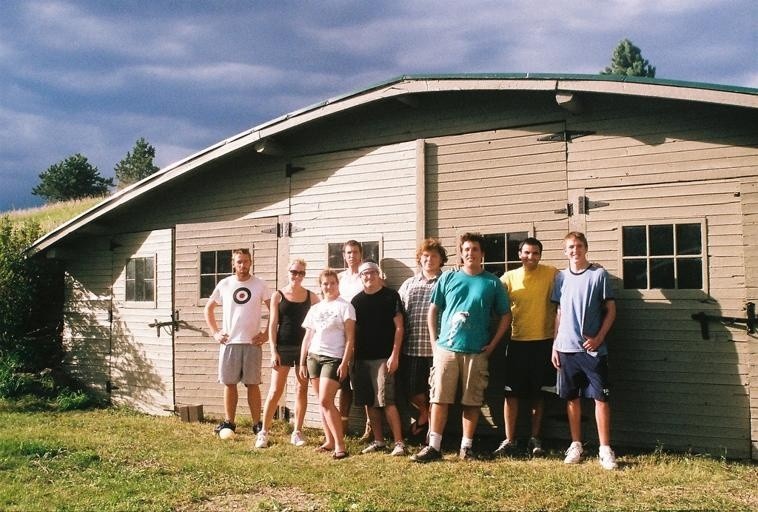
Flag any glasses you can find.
[363,272,377,276]
[290,269,307,279]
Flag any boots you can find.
[360,423,375,444]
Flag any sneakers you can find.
[411,446,442,464]
[599,445,618,470]
[527,437,543,455]
[461,445,475,462]
[393,441,407,457]
[288,431,307,447]
[253,422,263,433]
[494,439,516,455]
[256,429,268,448]
[360,441,386,455]
[565,441,584,463]
[222,421,235,432]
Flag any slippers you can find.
[410,419,428,435]
[334,451,350,458]
[314,445,334,452]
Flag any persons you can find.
[203,249,272,433]
[299,269,357,460]
[336,239,374,446]
[397,235,460,446]
[254,258,320,448]
[410,230,513,463]
[550,231,619,471]
[490,237,603,458]
[349,258,408,456]
